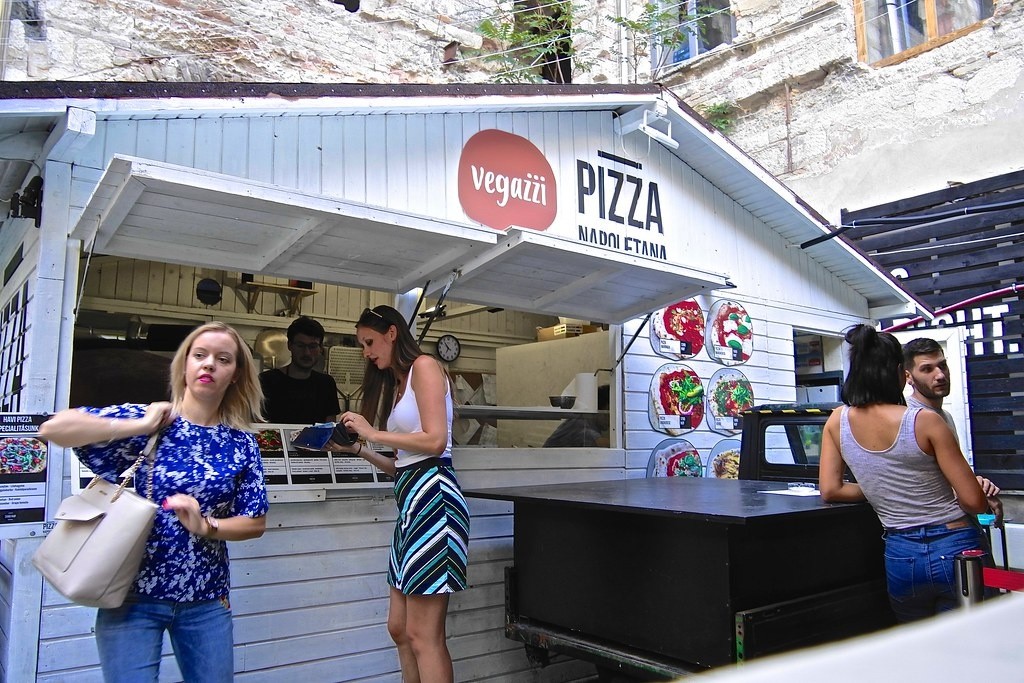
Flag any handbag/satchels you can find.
[28,430,163,609]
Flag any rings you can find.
[327,446,333,451]
[352,416,355,423]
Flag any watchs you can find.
[204,517,219,539]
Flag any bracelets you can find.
[355,443,363,456]
[108,417,119,446]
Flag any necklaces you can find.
[286,364,290,375]
[397,392,402,397]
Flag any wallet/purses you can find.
[291,420,360,453]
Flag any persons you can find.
[818,325,1000,625]
[322,305,470,683]
[541,385,610,448]
[257,315,342,424]
[40,320,268,683]
[902,338,1000,499]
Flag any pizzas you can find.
[652,299,753,478]
[255,430,283,448]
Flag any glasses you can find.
[290,341,319,352]
[358,308,383,320]
